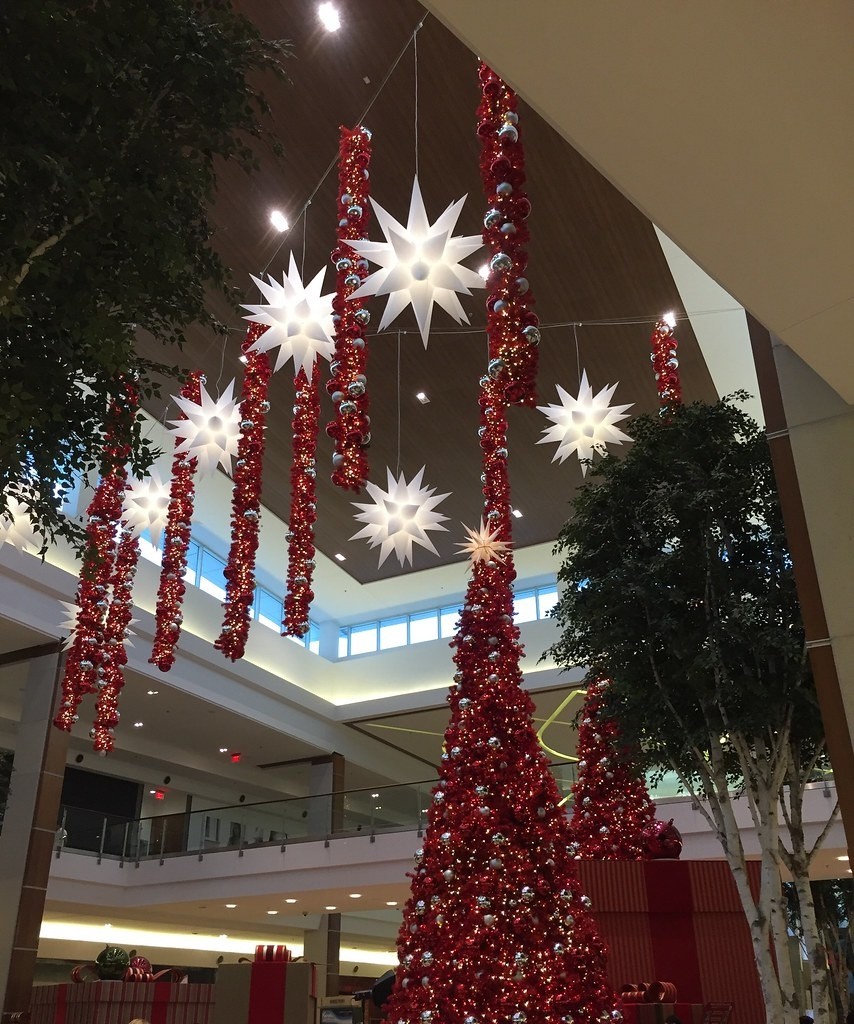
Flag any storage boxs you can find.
[212,963,319,1024]
[621,1000,708,1024]
[29,980,217,1024]
[574,852,787,1024]
